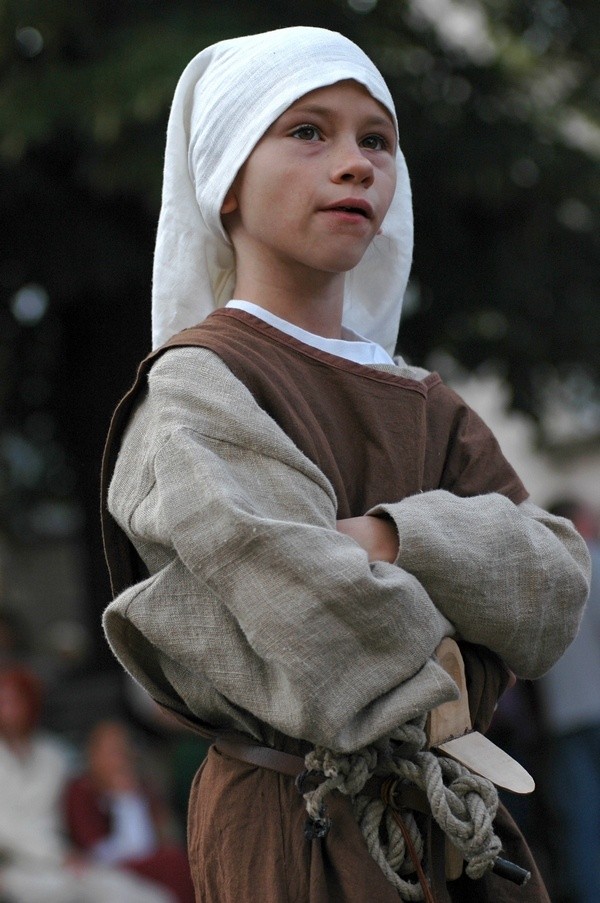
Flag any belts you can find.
[214,736,431,815]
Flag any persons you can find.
[100,25,592,902]
[1,651,198,903]
